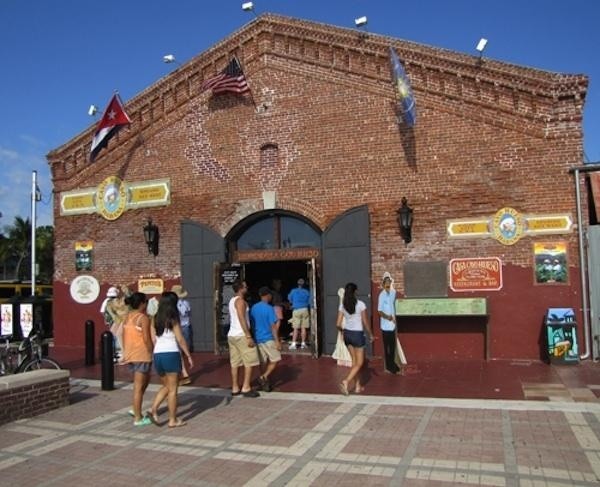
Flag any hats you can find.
[169,285,189,299]
[382,277,392,283]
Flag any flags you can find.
[389,46,417,125]
[201,56,250,96]
[90,92,132,153]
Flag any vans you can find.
[0,282,53,303]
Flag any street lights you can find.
[31,169,41,296]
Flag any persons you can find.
[336,282,375,394]
[288,279,311,349]
[172,285,191,385]
[377,272,401,376]
[228,279,283,397]
[148,291,194,427]
[100,281,134,363]
[123,292,153,426]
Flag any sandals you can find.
[337,380,350,396]
[232,387,258,397]
[353,383,366,394]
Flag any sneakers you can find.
[131,416,148,426]
[180,378,191,386]
[301,341,308,350]
[255,376,274,392]
[288,341,298,350]
[126,407,137,415]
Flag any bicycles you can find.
[0,322,61,374]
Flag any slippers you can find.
[149,408,162,426]
[168,420,186,429]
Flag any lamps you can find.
[395,196,415,244]
[142,214,161,256]
